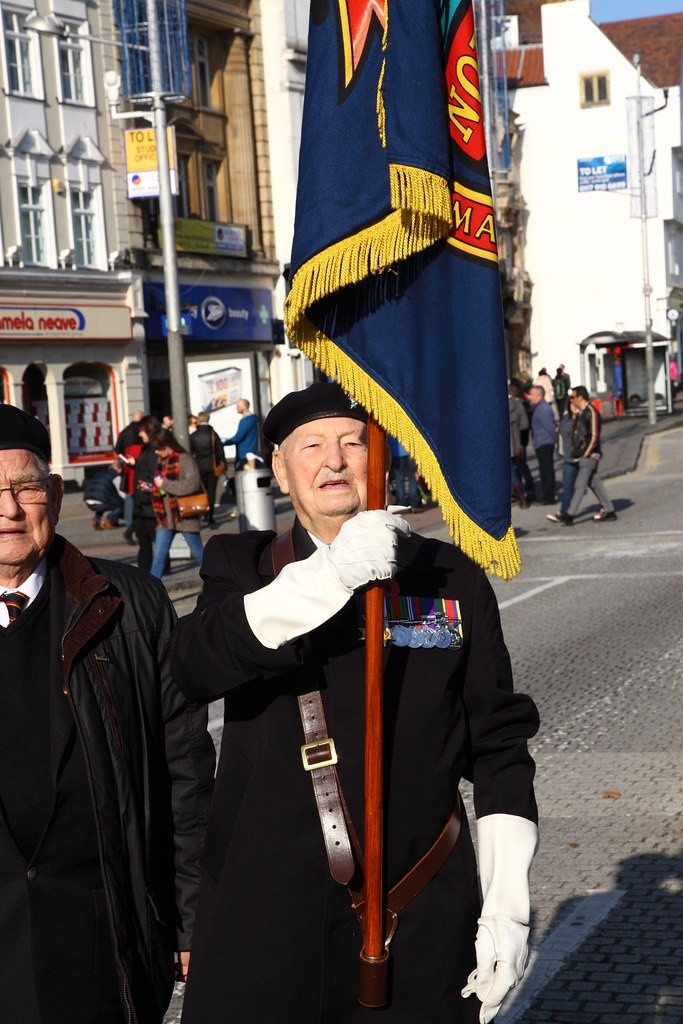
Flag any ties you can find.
[0,591,30,626]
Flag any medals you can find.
[358,620,464,652]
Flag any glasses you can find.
[569,395,577,399]
[0,472,53,504]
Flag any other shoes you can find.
[122,528,136,545]
[547,512,573,526]
[92,518,116,530]
[594,511,617,522]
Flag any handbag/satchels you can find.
[214,461,225,477]
[176,494,209,517]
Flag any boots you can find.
[512,481,528,509]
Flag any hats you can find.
[0,403,51,463]
[261,382,368,446]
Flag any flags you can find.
[282,0,522,584]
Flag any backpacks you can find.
[552,376,566,400]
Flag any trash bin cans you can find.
[235,470,276,534]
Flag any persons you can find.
[501,362,619,527]
[1,404,218,1024]
[385,433,437,513]
[168,382,542,1024]
[81,392,268,574]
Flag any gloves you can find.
[243,509,412,649]
[474,813,538,1008]
